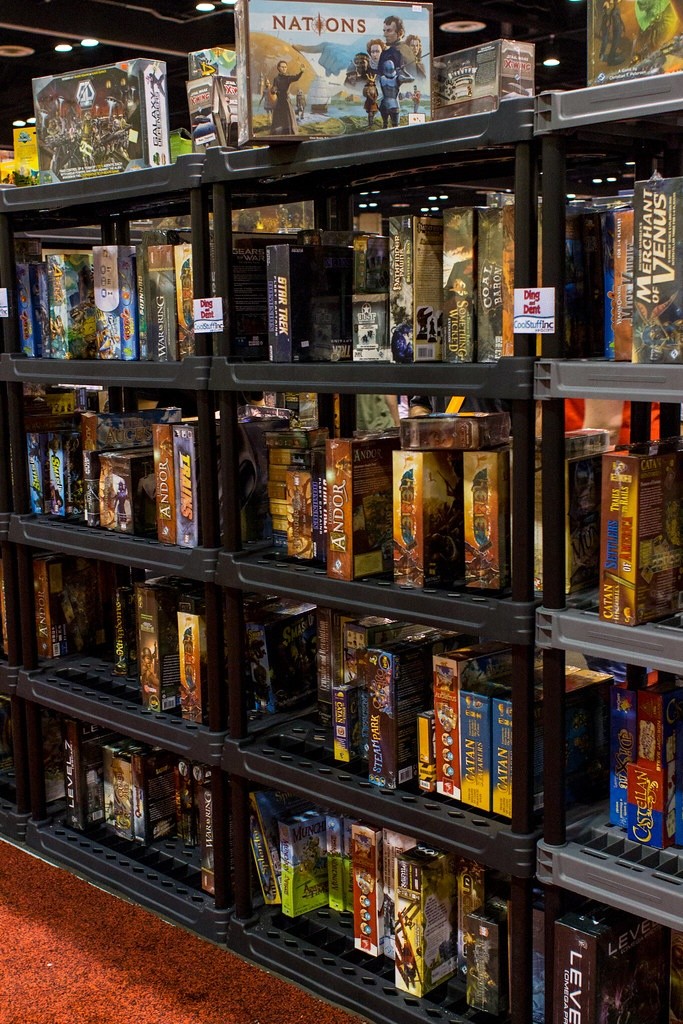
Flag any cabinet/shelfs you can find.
[537,73,683,1024]
[0,137,336,940]
[206,89,643,1024]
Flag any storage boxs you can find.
[0,0,683,1024]
